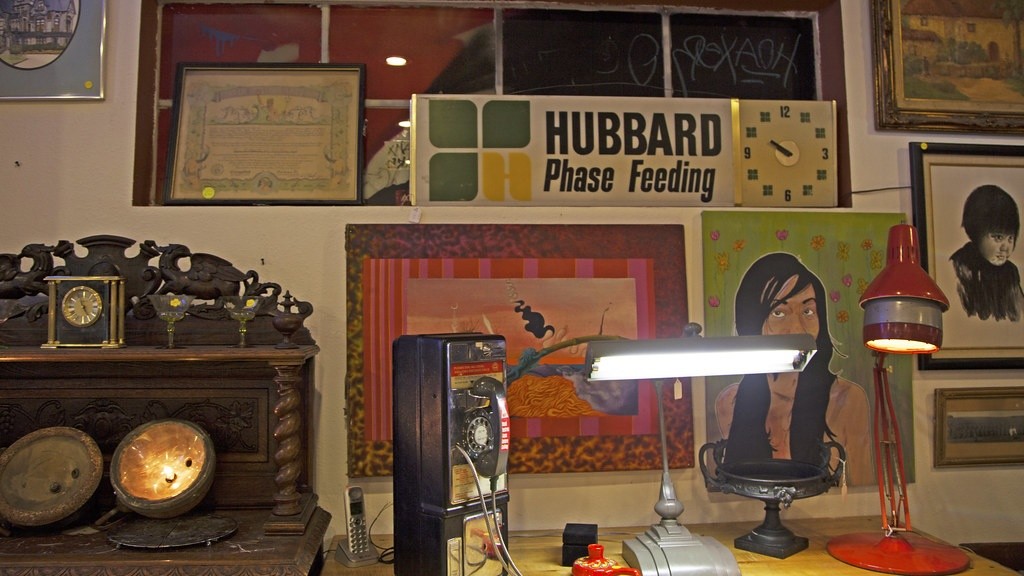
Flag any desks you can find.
[321,514,1020,576]
[0,506,333,576]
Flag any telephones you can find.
[334,486,379,568]
[393,331,510,517]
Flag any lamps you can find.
[582,333,817,576]
[826,223,972,576]
[61,286,101,328]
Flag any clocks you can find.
[731,99,838,208]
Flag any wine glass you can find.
[220,296,263,347]
[147,295,197,348]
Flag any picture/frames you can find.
[0,0,106,101]
[343,223,695,477]
[869,0,1024,137]
[933,386,1024,469]
[908,141,1024,371]
[162,61,367,206]
